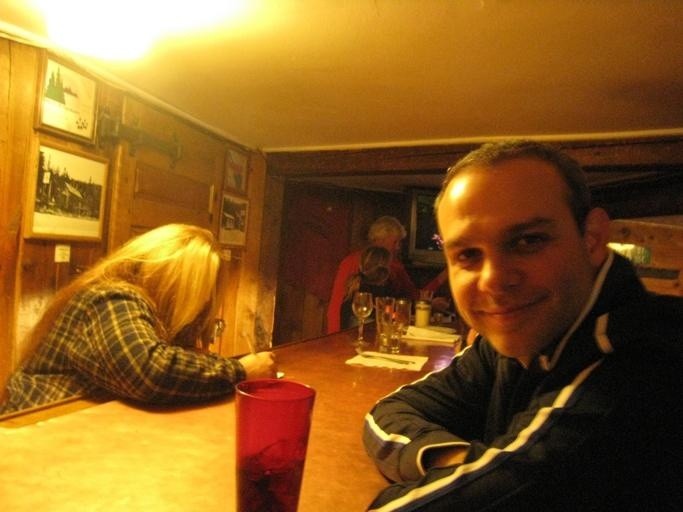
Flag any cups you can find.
[235,376,316,512]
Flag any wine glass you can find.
[375,297,411,354]
[351,292,373,349]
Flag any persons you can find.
[338,244,452,333]
[325,216,447,340]
[1,221,278,423]
[360,136,683,512]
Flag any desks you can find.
[3,316,463,512]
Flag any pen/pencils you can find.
[243,332,255,354]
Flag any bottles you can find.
[415,300,432,328]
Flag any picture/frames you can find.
[22,50,114,244]
[399,185,446,270]
[211,143,253,251]
